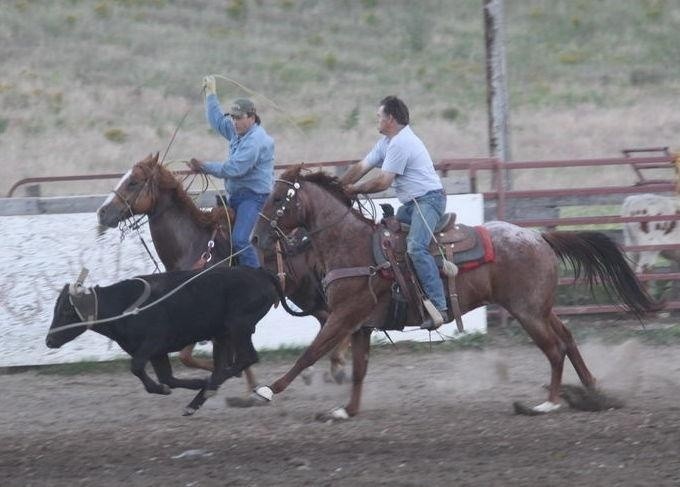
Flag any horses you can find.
[246,159,668,421]
[95,148,348,387]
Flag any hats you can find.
[223,99,257,117]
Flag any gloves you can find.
[203,76,217,96]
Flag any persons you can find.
[189,73,277,270]
[338,94,449,327]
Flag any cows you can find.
[618,191,680,292]
[44,264,311,418]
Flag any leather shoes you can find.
[421,311,448,329]
